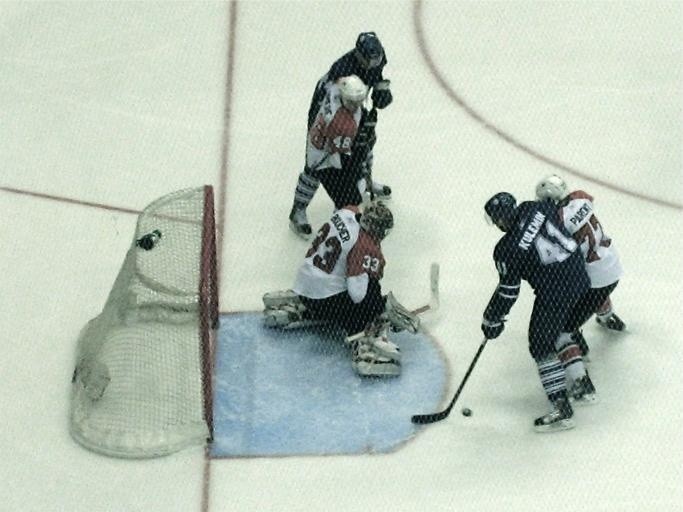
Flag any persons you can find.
[309,32,392,197]
[536,174,626,362]
[262,201,420,376]
[481,192,599,433]
[289,75,376,240]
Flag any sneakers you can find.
[368,178,392,195]
[291,210,312,235]
[356,344,401,366]
[597,313,626,331]
[267,308,290,325]
[566,373,596,396]
[533,397,573,425]
[573,333,591,356]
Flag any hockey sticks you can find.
[344,261,441,344]
[410,335,489,426]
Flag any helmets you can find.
[484,192,517,231]
[356,31,384,70]
[536,174,570,206]
[355,199,396,243]
[337,73,368,103]
